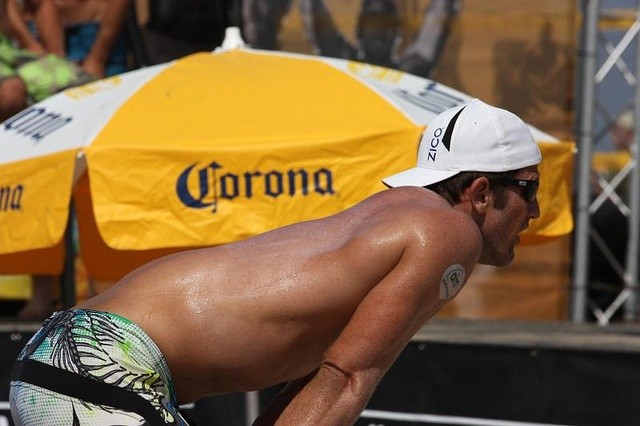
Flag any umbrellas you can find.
[1,25,576,426]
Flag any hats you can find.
[381,98,541,187]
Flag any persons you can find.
[9,96,542,426]
[0,0,242,119]
[303,0,463,77]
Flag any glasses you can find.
[498,178,539,203]
